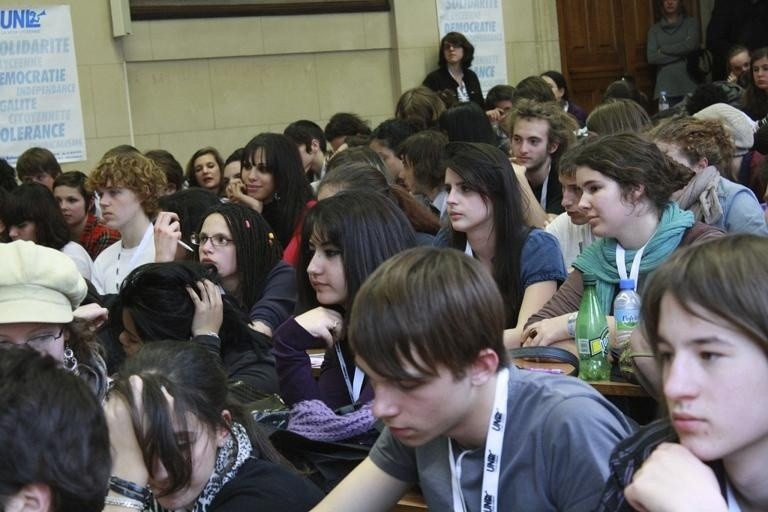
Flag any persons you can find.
[273,190,417,414]
[595,233,768,512]
[509,0,767,358]
[313,248,632,512]
[104,340,326,511]
[423,32,483,105]
[1,341,111,511]
[1,240,114,405]
[109,261,281,437]
[1,84,568,353]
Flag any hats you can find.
[690,102,756,157]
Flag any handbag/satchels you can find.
[247,418,376,496]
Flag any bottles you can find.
[656,90,670,114]
[613,279,644,380]
[574,272,612,384]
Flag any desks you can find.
[501,315,661,399]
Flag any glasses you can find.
[2,326,66,351]
[197,233,234,247]
[442,43,463,51]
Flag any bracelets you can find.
[104,476,152,510]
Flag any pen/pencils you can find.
[499,110,503,114]
[177,239,195,254]
